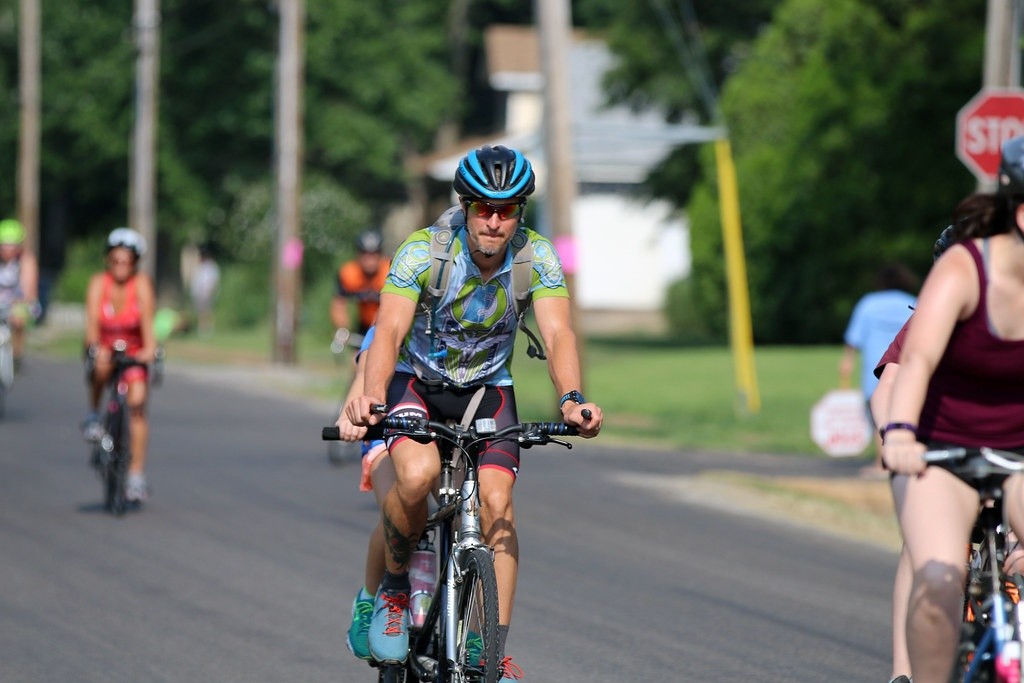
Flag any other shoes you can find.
[83,417,104,440]
[124,472,148,501]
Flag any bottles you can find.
[408,532,437,631]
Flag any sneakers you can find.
[479,655,524,683]
[467,630,486,669]
[347,587,376,661]
[371,584,411,666]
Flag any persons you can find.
[331,226,392,358]
[839,261,919,475]
[153,306,189,342]
[338,146,602,683]
[82,225,155,508]
[871,132,1024,683]
[0,218,34,376]
[179,246,220,333]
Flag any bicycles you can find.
[81,347,165,518]
[879,445,1024,683]
[323,400,594,683]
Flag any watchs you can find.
[559,389,585,416]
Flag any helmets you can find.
[998,136,1024,187]
[109,227,147,259]
[356,229,384,252]
[454,146,535,201]
[0,220,27,244]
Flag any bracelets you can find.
[885,421,918,435]
